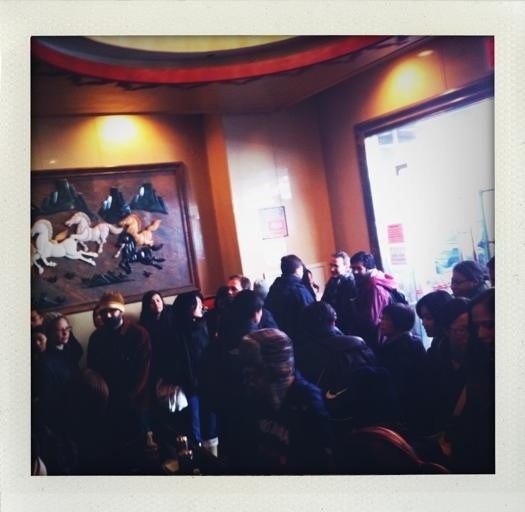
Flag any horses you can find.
[31,211,167,275]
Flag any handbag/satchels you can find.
[375,283,408,304]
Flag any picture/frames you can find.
[30,160,201,323]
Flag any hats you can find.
[42,312,63,330]
[99,290,125,312]
